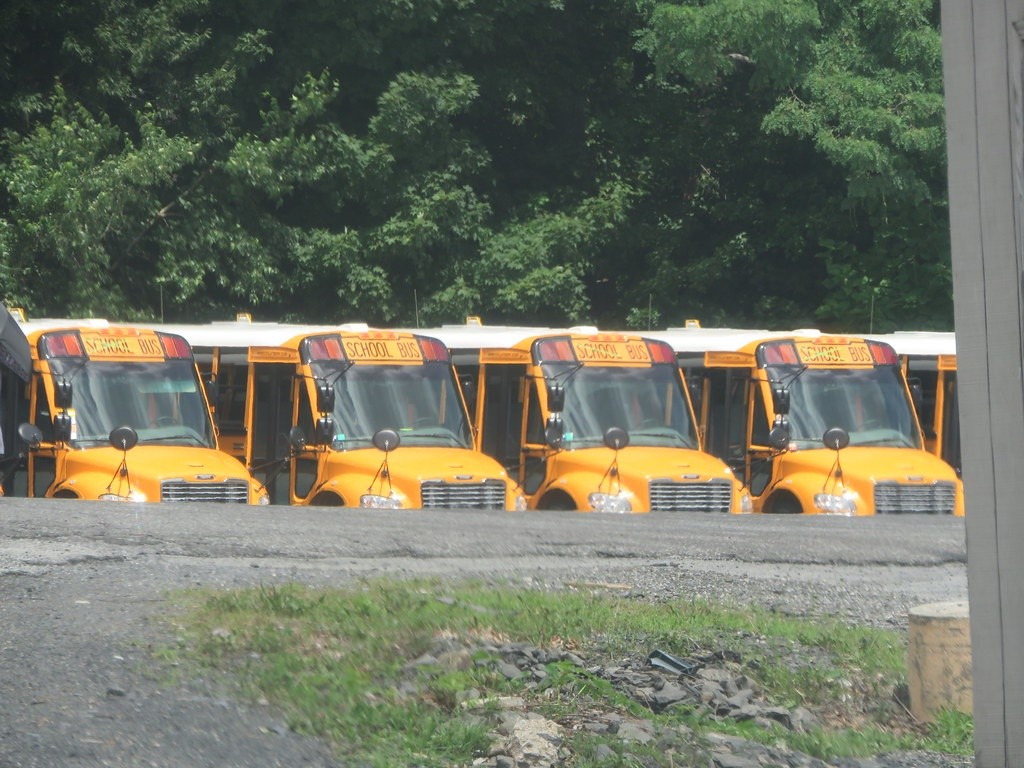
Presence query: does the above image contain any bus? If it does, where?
[835,353,963,484]
[456,331,789,514]
[664,333,964,516]
[194,330,564,513]
[21,326,308,508]
